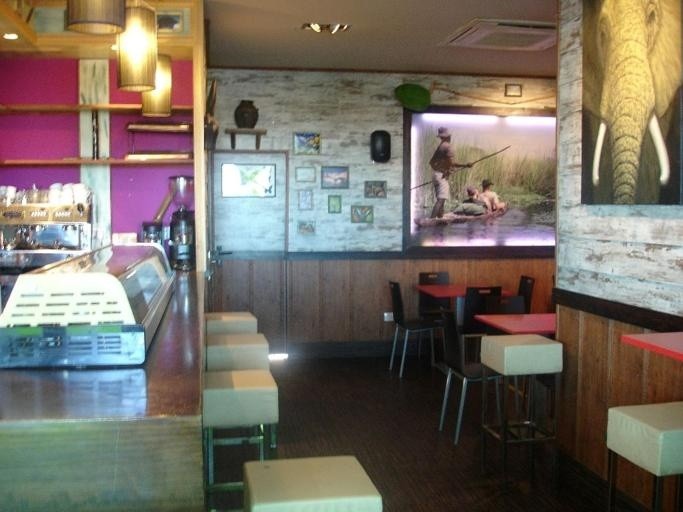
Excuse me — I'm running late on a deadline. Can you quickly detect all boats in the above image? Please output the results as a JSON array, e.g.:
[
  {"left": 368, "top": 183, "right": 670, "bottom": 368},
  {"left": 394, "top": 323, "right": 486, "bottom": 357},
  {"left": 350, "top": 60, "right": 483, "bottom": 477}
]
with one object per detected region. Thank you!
[{"left": 414, "top": 201, "right": 509, "bottom": 228}]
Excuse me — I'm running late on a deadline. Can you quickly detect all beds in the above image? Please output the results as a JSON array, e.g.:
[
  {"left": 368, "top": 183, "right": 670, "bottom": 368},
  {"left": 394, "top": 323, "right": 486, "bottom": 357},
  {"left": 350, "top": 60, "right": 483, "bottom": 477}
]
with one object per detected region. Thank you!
[{"left": 480, "top": 292, "right": 529, "bottom": 419}]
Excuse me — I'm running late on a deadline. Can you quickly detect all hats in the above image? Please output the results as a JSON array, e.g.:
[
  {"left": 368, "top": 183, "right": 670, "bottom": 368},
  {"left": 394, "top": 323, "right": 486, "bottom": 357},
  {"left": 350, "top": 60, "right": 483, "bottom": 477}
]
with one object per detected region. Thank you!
[
  {"left": 483, "top": 179, "right": 493, "bottom": 187},
  {"left": 437, "top": 127, "right": 451, "bottom": 138},
  {"left": 467, "top": 186, "right": 475, "bottom": 194}
]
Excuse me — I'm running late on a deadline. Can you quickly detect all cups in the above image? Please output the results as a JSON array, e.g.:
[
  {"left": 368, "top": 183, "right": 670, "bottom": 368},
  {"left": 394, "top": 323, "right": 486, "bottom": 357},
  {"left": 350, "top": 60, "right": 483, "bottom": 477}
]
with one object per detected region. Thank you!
[
  {"left": 0, "top": 185, "right": 16, "bottom": 204},
  {"left": 48, "top": 182, "right": 88, "bottom": 208}
]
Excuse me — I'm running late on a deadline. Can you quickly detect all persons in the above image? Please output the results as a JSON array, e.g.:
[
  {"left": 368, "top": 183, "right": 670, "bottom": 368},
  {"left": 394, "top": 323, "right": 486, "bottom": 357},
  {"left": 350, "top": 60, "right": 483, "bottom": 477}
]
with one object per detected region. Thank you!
[
  {"left": 425, "top": 125, "right": 473, "bottom": 218},
  {"left": 480, "top": 179, "right": 509, "bottom": 211},
  {"left": 461, "top": 185, "right": 488, "bottom": 214}
]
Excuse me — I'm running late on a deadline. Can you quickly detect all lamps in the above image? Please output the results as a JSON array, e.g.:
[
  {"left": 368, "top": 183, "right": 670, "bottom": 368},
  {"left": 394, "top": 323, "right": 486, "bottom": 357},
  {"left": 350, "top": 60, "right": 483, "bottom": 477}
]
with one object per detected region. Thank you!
[
  {"left": 304, "top": 22, "right": 352, "bottom": 34},
  {"left": 62, "top": 0, "right": 129, "bottom": 37},
  {"left": 139, "top": 50, "right": 175, "bottom": 120},
  {"left": 114, "top": 6, "right": 157, "bottom": 95}
]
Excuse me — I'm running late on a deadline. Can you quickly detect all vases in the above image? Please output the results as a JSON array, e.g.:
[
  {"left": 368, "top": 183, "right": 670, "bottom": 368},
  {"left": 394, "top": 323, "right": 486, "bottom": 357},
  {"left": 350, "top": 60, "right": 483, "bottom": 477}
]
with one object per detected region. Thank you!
[{"left": 232, "top": 99, "right": 258, "bottom": 127}]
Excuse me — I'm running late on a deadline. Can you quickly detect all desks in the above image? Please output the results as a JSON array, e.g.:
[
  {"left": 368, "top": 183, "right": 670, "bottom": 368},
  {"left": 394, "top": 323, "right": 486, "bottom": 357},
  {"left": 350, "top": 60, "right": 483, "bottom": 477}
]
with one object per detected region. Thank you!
[
  {"left": 614, "top": 329, "right": 682, "bottom": 367},
  {"left": 475, "top": 310, "right": 559, "bottom": 446}
]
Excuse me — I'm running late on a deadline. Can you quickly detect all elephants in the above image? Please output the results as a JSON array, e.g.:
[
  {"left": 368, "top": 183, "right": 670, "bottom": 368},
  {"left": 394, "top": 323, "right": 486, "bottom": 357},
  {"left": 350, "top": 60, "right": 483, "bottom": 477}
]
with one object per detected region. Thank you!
[{"left": 584, "top": 0, "right": 680, "bottom": 205}]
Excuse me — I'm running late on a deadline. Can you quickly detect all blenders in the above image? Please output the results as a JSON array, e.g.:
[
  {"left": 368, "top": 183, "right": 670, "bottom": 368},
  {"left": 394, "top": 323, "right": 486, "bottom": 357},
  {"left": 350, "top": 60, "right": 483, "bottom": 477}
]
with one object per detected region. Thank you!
[
  {"left": 140, "top": 220, "right": 162, "bottom": 247},
  {"left": 167, "top": 173, "right": 195, "bottom": 272}
]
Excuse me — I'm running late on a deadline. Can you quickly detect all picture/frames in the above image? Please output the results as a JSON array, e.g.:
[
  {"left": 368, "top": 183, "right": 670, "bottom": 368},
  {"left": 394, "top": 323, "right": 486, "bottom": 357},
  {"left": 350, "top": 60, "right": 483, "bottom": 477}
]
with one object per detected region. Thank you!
[
  {"left": 153, "top": 9, "right": 183, "bottom": 33},
  {"left": 291, "top": 132, "right": 323, "bottom": 155},
  {"left": 294, "top": 166, "right": 317, "bottom": 184},
  {"left": 319, "top": 166, "right": 350, "bottom": 189},
  {"left": 295, "top": 188, "right": 315, "bottom": 212},
  {"left": 218, "top": 161, "right": 277, "bottom": 198},
  {"left": 362, "top": 180, "right": 388, "bottom": 199},
  {"left": 349, "top": 204, "right": 374, "bottom": 224},
  {"left": 297, "top": 219, "right": 317, "bottom": 236},
  {"left": 504, "top": 82, "right": 523, "bottom": 97},
  {"left": 326, "top": 194, "right": 341, "bottom": 214},
  {"left": 399, "top": 104, "right": 558, "bottom": 259}
]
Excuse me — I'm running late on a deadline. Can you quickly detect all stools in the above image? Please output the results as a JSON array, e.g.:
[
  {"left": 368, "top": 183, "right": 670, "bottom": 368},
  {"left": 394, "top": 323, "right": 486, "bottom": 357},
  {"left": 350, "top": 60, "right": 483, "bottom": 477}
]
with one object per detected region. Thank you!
[
  {"left": 201, "top": 328, "right": 272, "bottom": 373},
  {"left": 599, "top": 391, "right": 682, "bottom": 511},
  {"left": 472, "top": 331, "right": 566, "bottom": 494},
  {"left": 236, "top": 452, "right": 385, "bottom": 512},
  {"left": 203, "top": 307, "right": 262, "bottom": 339},
  {"left": 197, "top": 364, "right": 281, "bottom": 512}
]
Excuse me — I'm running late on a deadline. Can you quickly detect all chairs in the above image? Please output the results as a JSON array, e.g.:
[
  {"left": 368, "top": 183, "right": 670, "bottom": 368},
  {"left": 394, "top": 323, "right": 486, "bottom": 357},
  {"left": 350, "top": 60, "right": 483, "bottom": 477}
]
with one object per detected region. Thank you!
[
  {"left": 380, "top": 272, "right": 448, "bottom": 379},
  {"left": 414, "top": 269, "right": 456, "bottom": 369},
  {"left": 433, "top": 299, "right": 522, "bottom": 444},
  {"left": 456, "top": 284, "right": 502, "bottom": 372},
  {"left": 503, "top": 276, "right": 536, "bottom": 317}
]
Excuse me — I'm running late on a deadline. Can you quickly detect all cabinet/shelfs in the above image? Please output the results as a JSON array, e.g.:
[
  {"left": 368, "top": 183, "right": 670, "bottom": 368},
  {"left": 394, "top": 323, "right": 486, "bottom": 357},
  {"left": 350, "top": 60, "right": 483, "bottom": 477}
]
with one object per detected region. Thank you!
[{"left": 0, "top": 98, "right": 197, "bottom": 174}]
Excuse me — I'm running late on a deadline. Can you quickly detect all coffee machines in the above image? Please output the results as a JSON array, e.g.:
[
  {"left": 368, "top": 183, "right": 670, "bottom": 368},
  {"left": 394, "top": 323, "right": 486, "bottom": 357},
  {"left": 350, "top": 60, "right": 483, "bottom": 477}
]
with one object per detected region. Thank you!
[{"left": 0, "top": 202, "right": 91, "bottom": 316}]
